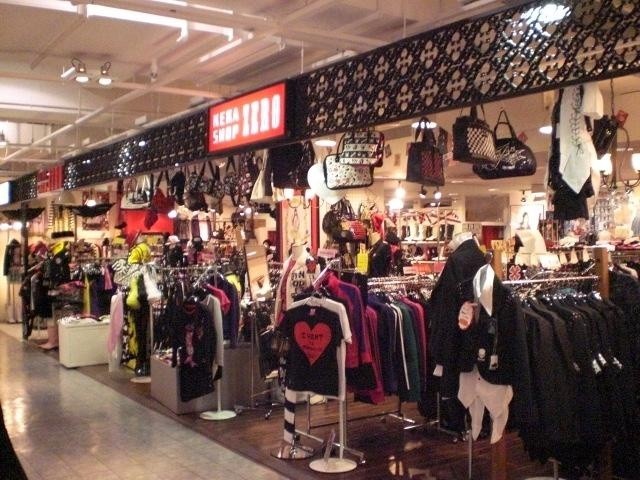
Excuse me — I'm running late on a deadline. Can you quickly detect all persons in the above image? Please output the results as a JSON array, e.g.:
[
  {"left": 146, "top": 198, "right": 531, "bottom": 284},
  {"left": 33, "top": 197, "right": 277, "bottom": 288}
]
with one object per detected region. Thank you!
[
  {"left": 273, "top": 242, "right": 315, "bottom": 366},
  {"left": 426, "top": 232, "right": 485, "bottom": 434},
  {"left": 366, "top": 233, "right": 392, "bottom": 279}
]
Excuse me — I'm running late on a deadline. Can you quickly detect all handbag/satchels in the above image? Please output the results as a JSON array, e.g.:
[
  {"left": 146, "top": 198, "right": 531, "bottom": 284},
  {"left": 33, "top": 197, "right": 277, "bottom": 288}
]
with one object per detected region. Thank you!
[
  {"left": 290, "top": 111, "right": 385, "bottom": 206},
  {"left": 474, "top": 110, "right": 537, "bottom": 180},
  {"left": 120, "top": 156, "right": 286, "bottom": 212},
  {"left": 592, "top": 114, "right": 618, "bottom": 156},
  {"left": 323, "top": 197, "right": 368, "bottom": 244},
  {"left": 406, "top": 117, "right": 445, "bottom": 188},
  {"left": 452, "top": 88, "right": 498, "bottom": 166}
]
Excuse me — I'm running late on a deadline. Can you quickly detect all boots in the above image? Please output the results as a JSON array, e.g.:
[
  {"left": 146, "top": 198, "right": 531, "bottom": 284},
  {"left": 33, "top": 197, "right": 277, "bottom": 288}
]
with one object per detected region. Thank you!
[{"left": 401, "top": 224, "right": 454, "bottom": 242}]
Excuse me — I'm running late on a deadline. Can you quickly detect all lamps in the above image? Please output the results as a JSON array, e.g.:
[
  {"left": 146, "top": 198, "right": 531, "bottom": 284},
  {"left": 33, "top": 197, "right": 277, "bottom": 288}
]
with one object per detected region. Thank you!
[{"left": 60, "top": 57, "right": 113, "bottom": 86}]
